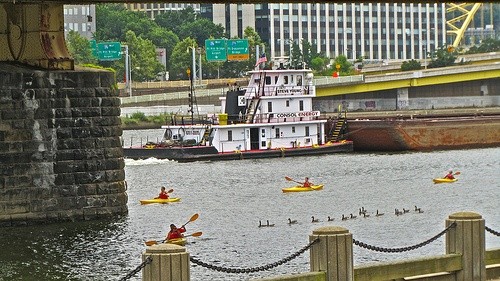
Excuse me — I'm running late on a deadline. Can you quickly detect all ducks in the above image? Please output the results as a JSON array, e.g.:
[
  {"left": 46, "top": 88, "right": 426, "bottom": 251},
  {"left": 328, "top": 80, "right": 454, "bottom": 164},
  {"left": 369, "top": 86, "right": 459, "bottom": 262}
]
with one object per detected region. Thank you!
[{"left": 257, "top": 205, "right": 424, "bottom": 228}]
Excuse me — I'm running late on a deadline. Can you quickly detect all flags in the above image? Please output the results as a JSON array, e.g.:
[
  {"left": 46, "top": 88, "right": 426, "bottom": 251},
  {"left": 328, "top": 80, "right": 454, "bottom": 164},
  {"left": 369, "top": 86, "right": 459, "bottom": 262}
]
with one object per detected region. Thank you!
[{"left": 255, "top": 53, "right": 267, "bottom": 66}]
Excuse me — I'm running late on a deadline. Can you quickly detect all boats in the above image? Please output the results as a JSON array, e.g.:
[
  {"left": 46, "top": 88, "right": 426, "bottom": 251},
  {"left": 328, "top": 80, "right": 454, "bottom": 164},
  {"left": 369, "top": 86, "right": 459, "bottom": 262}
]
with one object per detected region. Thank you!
[
  {"left": 145, "top": 238, "right": 187, "bottom": 246},
  {"left": 122, "top": 61, "right": 353, "bottom": 162},
  {"left": 433, "top": 178, "right": 459, "bottom": 184},
  {"left": 139, "top": 197, "right": 181, "bottom": 205},
  {"left": 281, "top": 183, "right": 324, "bottom": 192}
]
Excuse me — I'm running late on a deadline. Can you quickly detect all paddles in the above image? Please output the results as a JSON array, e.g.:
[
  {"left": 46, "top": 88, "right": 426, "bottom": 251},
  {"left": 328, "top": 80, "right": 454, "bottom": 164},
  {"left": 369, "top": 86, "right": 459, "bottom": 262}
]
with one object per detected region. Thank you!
[
  {"left": 164, "top": 213, "right": 199, "bottom": 240},
  {"left": 145, "top": 231, "right": 202, "bottom": 246},
  {"left": 154, "top": 189, "right": 173, "bottom": 199},
  {"left": 284, "top": 176, "right": 304, "bottom": 185},
  {"left": 446, "top": 172, "right": 460, "bottom": 179}
]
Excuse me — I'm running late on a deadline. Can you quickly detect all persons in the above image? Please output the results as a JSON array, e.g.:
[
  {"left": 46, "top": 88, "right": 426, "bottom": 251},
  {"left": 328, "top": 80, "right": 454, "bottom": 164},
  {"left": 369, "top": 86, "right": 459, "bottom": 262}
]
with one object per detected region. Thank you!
[
  {"left": 302, "top": 177, "right": 312, "bottom": 187},
  {"left": 166, "top": 224, "right": 187, "bottom": 241},
  {"left": 445, "top": 170, "right": 455, "bottom": 179},
  {"left": 159, "top": 186, "right": 169, "bottom": 199}
]
